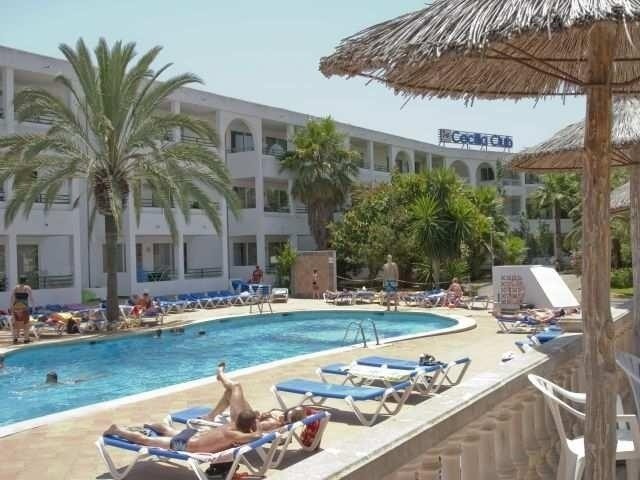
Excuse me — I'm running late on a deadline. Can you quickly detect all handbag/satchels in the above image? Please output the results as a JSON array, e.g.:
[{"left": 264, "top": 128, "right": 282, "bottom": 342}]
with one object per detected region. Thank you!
[{"left": 284, "top": 405, "right": 331, "bottom": 452}]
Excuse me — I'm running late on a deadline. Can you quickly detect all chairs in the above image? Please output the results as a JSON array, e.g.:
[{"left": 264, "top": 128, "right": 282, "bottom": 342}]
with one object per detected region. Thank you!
[
  {"left": 321, "top": 288, "right": 491, "bottom": 310},
  {"left": 98, "top": 423, "right": 280, "bottom": 479},
  {"left": 495, "top": 318, "right": 562, "bottom": 334},
  {"left": 0, "top": 274, "right": 290, "bottom": 340},
  {"left": 527, "top": 348, "right": 640, "bottom": 479},
  {"left": 320, "top": 362, "right": 445, "bottom": 402},
  {"left": 272, "top": 378, "right": 412, "bottom": 427},
  {"left": 172, "top": 406, "right": 332, "bottom": 468},
  {"left": 352, "top": 356, "right": 472, "bottom": 394}
]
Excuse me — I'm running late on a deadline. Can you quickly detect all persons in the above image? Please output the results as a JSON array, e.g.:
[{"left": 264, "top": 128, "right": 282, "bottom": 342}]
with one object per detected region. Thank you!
[
  {"left": 11, "top": 275, "right": 35, "bottom": 344},
  {"left": 45, "top": 372, "right": 90, "bottom": 385},
  {"left": 128, "top": 293, "right": 154, "bottom": 309},
  {"left": 311, "top": 269, "right": 320, "bottom": 299},
  {"left": 103, "top": 362, "right": 304, "bottom": 453},
  {"left": 247, "top": 266, "right": 263, "bottom": 283},
  {"left": 447, "top": 278, "right": 462, "bottom": 307},
  {"left": 384, "top": 254, "right": 399, "bottom": 311},
  {"left": 153, "top": 328, "right": 206, "bottom": 338},
  {"left": 492, "top": 308, "right": 565, "bottom": 325},
  {"left": 0, "top": 354, "right": 6, "bottom": 368}
]
